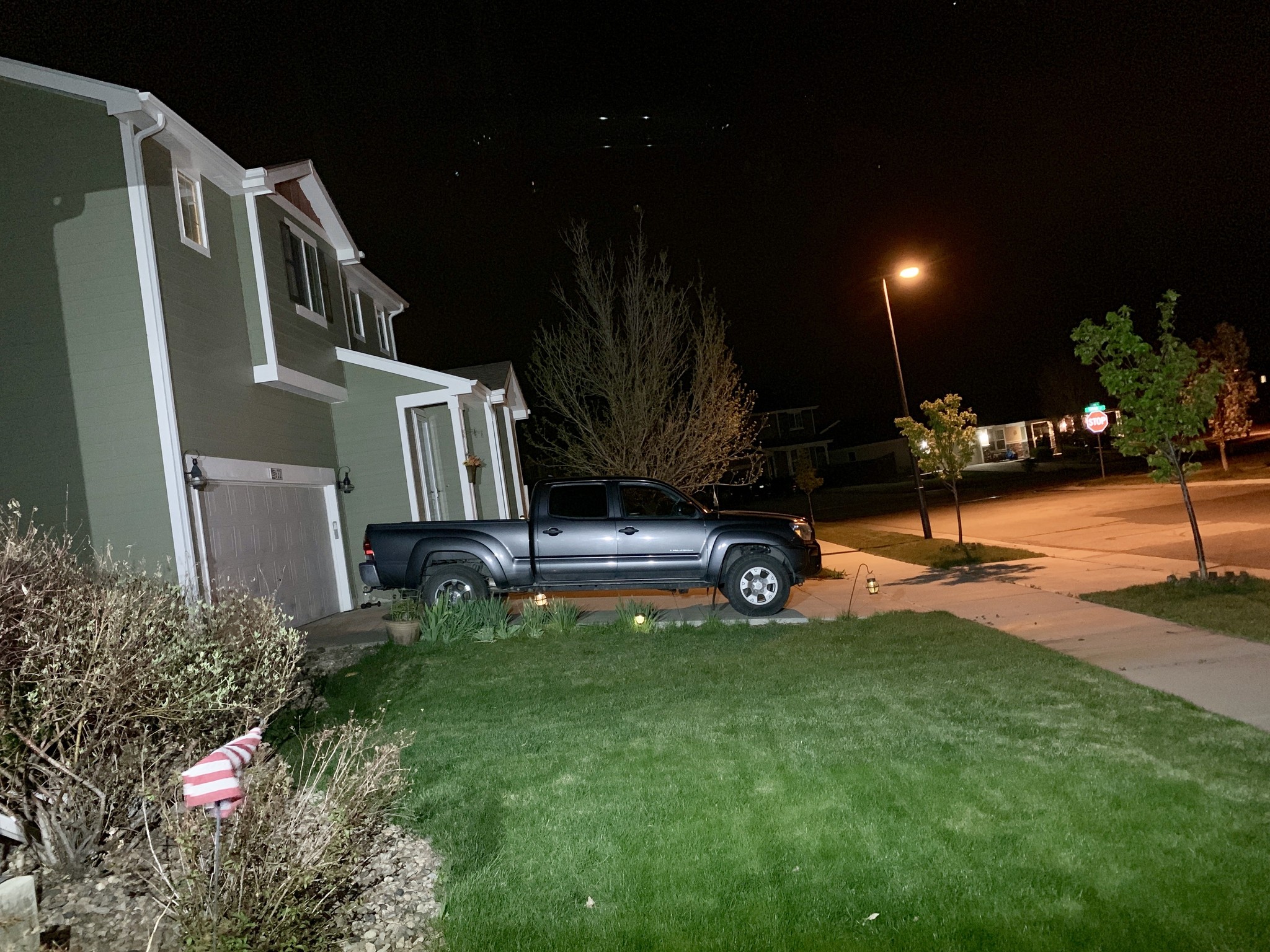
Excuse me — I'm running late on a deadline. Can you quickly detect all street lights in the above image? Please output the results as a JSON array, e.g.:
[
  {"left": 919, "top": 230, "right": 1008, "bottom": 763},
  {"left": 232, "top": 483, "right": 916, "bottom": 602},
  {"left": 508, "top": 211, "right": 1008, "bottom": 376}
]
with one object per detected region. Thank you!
[{"left": 881, "top": 267, "right": 932, "bottom": 539}]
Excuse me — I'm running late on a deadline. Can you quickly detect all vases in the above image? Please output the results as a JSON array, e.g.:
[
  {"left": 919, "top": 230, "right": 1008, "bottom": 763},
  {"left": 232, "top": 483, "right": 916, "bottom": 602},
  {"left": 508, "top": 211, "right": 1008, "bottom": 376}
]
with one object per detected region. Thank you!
[{"left": 465, "top": 465, "right": 476, "bottom": 483}]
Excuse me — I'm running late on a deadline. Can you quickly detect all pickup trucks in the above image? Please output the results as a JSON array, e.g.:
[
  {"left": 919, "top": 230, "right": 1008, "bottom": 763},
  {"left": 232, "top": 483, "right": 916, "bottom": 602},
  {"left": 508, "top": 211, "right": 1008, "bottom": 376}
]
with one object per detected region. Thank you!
[{"left": 358, "top": 476, "right": 822, "bottom": 616}]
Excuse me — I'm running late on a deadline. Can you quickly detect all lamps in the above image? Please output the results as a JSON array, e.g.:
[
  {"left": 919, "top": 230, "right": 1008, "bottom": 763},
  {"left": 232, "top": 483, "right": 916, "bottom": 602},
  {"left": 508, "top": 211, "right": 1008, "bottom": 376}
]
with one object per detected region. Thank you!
[
  {"left": 635, "top": 613, "right": 646, "bottom": 624},
  {"left": 336, "top": 466, "right": 354, "bottom": 494},
  {"left": 865, "top": 578, "right": 879, "bottom": 595},
  {"left": 183, "top": 448, "right": 208, "bottom": 489},
  {"left": 534, "top": 589, "right": 547, "bottom": 605}
]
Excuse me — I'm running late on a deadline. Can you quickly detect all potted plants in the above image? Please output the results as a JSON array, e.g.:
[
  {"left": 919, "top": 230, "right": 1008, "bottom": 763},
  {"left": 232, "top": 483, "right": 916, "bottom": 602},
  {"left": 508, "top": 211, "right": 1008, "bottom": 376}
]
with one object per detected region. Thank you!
[{"left": 382, "top": 599, "right": 420, "bottom": 643}]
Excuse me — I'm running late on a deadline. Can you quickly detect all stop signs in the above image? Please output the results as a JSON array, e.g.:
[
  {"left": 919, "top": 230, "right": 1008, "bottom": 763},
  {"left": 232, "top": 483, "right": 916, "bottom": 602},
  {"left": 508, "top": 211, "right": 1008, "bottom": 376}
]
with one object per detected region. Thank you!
[{"left": 1086, "top": 411, "right": 1109, "bottom": 433}]
